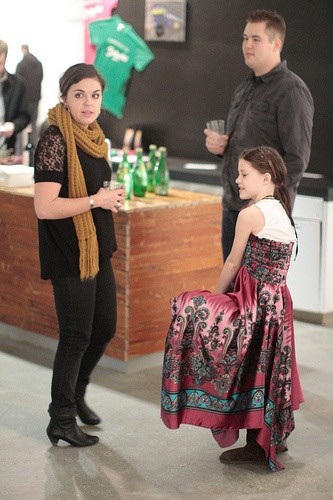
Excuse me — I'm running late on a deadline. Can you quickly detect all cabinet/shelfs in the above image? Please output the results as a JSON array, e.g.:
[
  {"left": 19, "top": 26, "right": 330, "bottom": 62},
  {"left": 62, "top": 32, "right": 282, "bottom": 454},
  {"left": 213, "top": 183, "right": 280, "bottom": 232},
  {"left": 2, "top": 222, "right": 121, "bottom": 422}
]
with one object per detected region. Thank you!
[{"left": 166, "top": 157, "right": 333, "bottom": 326}]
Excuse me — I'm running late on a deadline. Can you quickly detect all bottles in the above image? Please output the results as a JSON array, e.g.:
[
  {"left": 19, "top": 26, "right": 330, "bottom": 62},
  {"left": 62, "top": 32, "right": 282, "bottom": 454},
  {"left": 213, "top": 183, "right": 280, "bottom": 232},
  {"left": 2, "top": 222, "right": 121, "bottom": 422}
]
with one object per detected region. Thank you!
[
  {"left": 22, "top": 132, "right": 35, "bottom": 167},
  {"left": 115, "top": 147, "right": 131, "bottom": 200},
  {"left": 154, "top": 146, "right": 170, "bottom": 196},
  {"left": 147, "top": 145, "right": 158, "bottom": 193},
  {"left": 132, "top": 148, "right": 147, "bottom": 198}
]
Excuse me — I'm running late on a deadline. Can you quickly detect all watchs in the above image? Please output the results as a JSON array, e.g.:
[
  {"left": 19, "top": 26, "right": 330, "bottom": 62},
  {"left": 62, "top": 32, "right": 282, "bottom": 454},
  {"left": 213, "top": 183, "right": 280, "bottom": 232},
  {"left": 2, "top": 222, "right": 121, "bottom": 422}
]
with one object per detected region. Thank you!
[{"left": 89, "top": 196, "right": 94, "bottom": 209}]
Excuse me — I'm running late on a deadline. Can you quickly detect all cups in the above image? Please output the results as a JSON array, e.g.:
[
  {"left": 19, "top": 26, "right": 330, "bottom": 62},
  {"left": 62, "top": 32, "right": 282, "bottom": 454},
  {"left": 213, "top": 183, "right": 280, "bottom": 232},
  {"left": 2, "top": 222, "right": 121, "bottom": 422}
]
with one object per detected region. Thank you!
[
  {"left": 205, "top": 119, "right": 226, "bottom": 135},
  {"left": 103, "top": 181, "right": 122, "bottom": 210}
]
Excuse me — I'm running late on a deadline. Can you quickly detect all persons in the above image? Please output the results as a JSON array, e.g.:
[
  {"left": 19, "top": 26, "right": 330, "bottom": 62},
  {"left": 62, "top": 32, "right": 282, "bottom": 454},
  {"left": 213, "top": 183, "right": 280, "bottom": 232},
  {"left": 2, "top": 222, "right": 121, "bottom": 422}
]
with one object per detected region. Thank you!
[
  {"left": 203, "top": 9, "right": 314, "bottom": 265},
  {"left": 160, "top": 147, "right": 304, "bottom": 472},
  {"left": 0, "top": 40, "right": 43, "bottom": 157},
  {"left": 33, "top": 63, "right": 126, "bottom": 446}
]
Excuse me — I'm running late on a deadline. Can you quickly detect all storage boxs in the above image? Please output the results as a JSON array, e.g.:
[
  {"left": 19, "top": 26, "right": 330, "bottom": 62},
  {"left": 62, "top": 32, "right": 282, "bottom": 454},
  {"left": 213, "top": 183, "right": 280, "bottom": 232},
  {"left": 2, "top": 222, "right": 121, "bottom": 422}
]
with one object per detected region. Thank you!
[{"left": 0, "top": 165, "right": 34, "bottom": 187}]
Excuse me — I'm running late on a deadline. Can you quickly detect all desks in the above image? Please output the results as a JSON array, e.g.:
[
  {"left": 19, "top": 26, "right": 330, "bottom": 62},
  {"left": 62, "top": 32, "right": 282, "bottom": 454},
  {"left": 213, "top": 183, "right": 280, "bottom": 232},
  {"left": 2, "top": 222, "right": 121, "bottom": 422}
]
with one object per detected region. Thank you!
[{"left": 0, "top": 186, "right": 223, "bottom": 375}]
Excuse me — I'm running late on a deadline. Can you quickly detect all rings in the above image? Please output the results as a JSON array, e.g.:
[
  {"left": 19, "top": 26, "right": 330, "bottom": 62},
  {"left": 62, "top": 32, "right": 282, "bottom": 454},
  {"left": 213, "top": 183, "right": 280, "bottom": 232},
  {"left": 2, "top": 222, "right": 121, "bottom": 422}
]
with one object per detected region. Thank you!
[{"left": 116, "top": 203, "right": 121, "bottom": 208}]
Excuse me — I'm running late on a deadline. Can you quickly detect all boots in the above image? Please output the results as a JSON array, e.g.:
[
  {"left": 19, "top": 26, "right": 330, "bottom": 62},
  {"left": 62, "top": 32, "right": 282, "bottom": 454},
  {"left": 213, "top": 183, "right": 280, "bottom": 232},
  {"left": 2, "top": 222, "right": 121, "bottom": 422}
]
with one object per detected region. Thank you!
[
  {"left": 75, "top": 382, "right": 100, "bottom": 425},
  {"left": 46, "top": 402, "right": 98, "bottom": 447}
]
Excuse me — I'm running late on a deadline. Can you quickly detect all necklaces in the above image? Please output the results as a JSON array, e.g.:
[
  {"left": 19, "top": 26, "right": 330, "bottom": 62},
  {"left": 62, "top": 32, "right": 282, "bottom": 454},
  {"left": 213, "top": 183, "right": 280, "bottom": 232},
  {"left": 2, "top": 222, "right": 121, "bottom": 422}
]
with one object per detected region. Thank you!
[{"left": 262, "top": 195, "right": 274, "bottom": 199}]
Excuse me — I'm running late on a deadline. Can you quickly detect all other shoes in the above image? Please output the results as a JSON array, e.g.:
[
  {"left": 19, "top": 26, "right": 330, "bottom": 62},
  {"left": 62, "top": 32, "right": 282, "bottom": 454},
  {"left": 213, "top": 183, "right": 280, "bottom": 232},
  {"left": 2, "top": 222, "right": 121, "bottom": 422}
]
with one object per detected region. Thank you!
[{"left": 219, "top": 428, "right": 267, "bottom": 465}]
